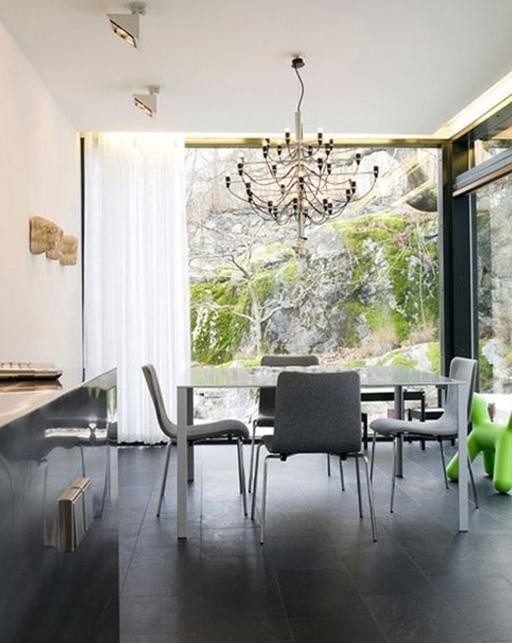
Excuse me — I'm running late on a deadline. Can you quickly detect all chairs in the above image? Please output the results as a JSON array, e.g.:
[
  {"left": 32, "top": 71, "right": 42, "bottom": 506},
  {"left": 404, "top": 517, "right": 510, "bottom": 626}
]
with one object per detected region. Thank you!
[
  {"left": 369, "top": 356, "right": 478, "bottom": 513},
  {"left": 250, "top": 371, "right": 378, "bottom": 545},
  {"left": 250, "top": 355, "right": 319, "bottom": 494},
  {"left": 142, "top": 364, "right": 249, "bottom": 518},
  {"left": 408, "top": 385, "right": 455, "bottom": 450}
]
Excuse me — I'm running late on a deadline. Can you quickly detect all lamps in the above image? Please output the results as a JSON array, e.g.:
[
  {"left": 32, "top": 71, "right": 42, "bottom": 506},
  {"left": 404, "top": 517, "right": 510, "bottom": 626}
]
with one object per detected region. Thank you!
[
  {"left": 134, "top": 86, "right": 158, "bottom": 115},
  {"left": 107, "top": 3, "right": 147, "bottom": 49},
  {"left": 226, "top": 57, "right": 377, "bottom": 255}
]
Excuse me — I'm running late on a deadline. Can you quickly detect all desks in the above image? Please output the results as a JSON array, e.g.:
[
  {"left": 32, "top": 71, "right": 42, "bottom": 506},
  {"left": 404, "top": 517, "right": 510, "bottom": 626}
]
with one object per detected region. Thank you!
[
  {"left": 175, "top": 364, "right": 469, "bottom": 539},
  {"left": 361, "top": 386, "right": 426, "bottom": 449}
]
